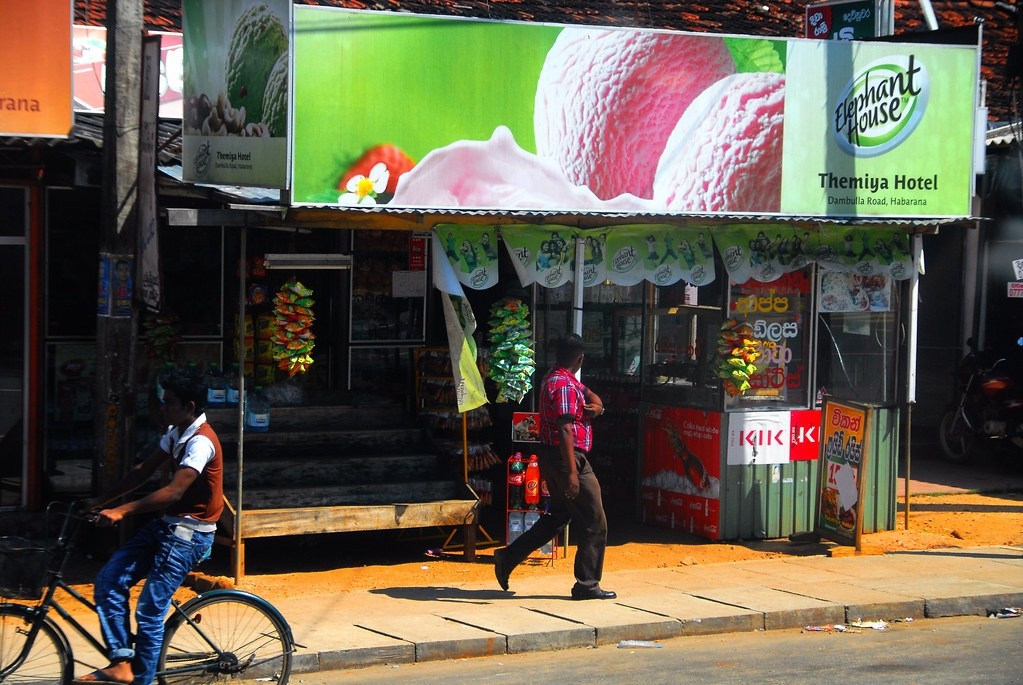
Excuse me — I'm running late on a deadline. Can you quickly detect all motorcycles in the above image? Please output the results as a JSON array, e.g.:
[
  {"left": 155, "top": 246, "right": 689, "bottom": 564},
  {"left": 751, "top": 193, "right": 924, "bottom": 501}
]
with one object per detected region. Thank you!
[{"left": 940, "top": 335, "right": 1023, "bottom": 462}]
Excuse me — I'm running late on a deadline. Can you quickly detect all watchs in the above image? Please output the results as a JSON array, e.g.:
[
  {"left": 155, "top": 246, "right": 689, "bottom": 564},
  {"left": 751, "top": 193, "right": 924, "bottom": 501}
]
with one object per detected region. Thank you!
[{"left": 598, "top": 404, "right": 605, "bottom": 416}]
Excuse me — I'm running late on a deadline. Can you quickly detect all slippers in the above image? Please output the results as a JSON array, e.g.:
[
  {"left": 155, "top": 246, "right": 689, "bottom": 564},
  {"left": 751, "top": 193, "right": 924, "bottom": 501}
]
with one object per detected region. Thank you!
[{"left": 72, "top": 670, "right": 126, "bottom": 685}]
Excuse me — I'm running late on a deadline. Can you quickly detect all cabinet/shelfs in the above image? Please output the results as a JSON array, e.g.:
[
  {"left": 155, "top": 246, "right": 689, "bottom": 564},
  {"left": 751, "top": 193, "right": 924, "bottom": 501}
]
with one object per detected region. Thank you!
[
  {"left": 198, "top": 400, "right": 482, "bottom": 585},
  {"left": 412, "top": 345, "right": 503, "bottom": 550}
]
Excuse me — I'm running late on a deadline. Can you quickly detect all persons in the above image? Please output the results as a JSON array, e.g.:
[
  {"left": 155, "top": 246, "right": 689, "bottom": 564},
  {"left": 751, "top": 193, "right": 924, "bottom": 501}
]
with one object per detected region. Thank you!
[
  {"left": 749, "top": 231, "right": 809, "bottom": 268},
  {"left": 535, "top": 233, "right": 576, "bottom": 272},
  {"left": 494, "top": 332, "right": 619, "bottom": 599},
  {"left": 695, "top": 233, "right": 713, "bottom": 259},
  {"left": 75, "top": 368, "right": 222, "bottom": 685},
  {"left": 677, "top": 238, "right": 699, "bottom": 270},
  {"left": 874, "top": 238, "right": 896, "bottom": 265},
  {"left": 460, "top": 240, "right": 480, "bottom": 273},
  {"left": 513, "top": 414, "right": 538, "bottom": 440},
  {"left": 892, "top": 232, "right": 910, "bottom": 257},
  {"left": 660, "top": 230, "right": 678, "bottom": 265},
  {"left": 641, "top": 235, "right": 660, "bottom": 265},
  {"left": 584, "top": 234, "right": 607, "bottom": 265},
  {"left": 857, "top": 230, "right": 875, "bottom": 260},
  {"left": 839, "top": 233, "right": 857, "bottom": 257},
  {"left": 478, "top": 233, "right": 497, "bottom": 262},
  {"left": 98, "top": 259, "right": 104, "bottom": 279},
  {"left": 114, "top": 261, "right": 131, "bottom": 282},
  {"left": 438, "top": 227, "right": 461, "bottom": 263}
]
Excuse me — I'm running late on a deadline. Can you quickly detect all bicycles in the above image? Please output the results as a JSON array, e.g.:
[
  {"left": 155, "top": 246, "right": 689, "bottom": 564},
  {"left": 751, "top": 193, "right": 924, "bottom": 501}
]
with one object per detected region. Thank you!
[{"left": 0, "top": 494, "right": 298, "bottom": 685}]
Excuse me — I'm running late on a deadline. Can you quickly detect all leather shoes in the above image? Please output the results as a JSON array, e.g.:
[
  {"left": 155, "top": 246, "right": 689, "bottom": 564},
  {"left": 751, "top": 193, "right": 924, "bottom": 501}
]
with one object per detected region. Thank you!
[
  {"left": 494, "top": 549, "right": 509, "bottom": 591},
  {"left": 571, "top": 583, "right": 617, "bottom": 601}
]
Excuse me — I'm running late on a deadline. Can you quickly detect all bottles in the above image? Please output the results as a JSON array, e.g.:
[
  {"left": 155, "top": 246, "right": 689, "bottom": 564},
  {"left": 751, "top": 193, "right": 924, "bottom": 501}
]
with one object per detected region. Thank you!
[
  {"left": 524, "top": 505, "right": 540, "bottom": 533},
  {"left": 206, "top": 362, "right": 225, "bottom": 408},
  {"left": 539, "top": 472, "right": 551, "bottom": 510},
  {"left": 244, "top": 385, "right": 270, "bottom": 431},
  {"left": 525, "top": 455, "right": 540, "bottom": 509},
  {"left": 540, "top": 537, "right": 555, "bottom": 555},
  {"left": 509, "top": 452, "right": 525, "bottom": 509},
  {"left": 157, "top": 362, "right": 196, "bottom": 404},
  {"left": 508, "top": 503, "right": 524, "bottom": 545},
  {"left": 225, "top": 363, "right": 247, "bottom": 408}
]
